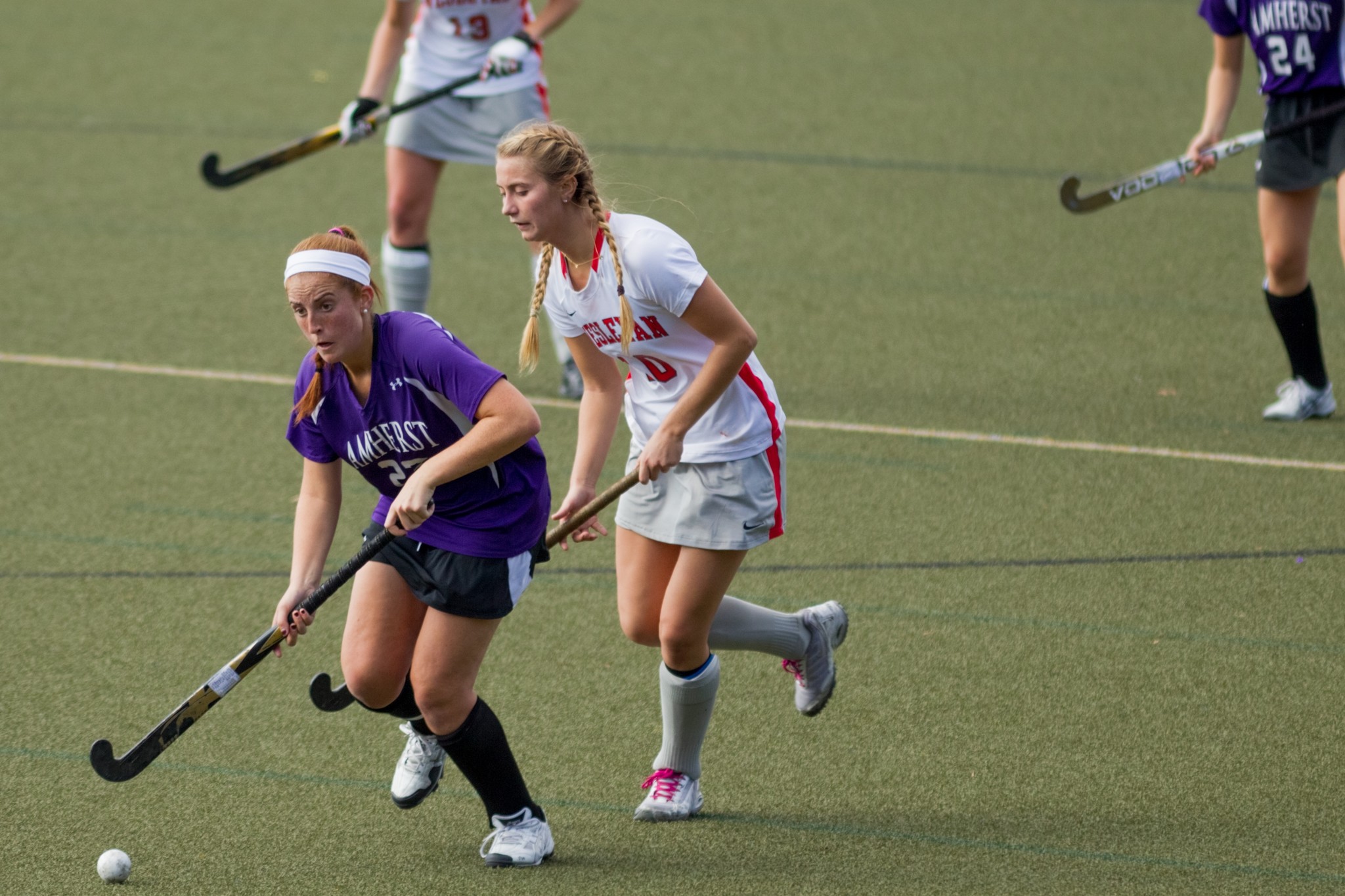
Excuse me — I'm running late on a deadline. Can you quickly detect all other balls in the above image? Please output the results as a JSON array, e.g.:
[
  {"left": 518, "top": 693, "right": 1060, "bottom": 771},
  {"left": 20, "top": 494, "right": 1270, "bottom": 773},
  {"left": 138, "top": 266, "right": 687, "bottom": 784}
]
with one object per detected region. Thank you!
[{"left": 97, "top": 848, "right": 132, "bottom": 883}]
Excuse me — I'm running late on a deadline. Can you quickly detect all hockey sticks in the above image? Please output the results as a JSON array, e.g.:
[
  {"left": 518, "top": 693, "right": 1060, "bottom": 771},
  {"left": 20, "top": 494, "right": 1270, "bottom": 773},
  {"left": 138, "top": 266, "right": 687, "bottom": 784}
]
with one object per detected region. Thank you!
[
  {"left": 306, "top": 463, "right": 643, "bottom": 714},
  {"left": 198, "top": 60, "right": 523, "bottom": 191},
  {"left": 1059, "top": 99, "right": 1345, "bottom": 217},
  {"left": 88, "top": 496, "right": 433, "bottom": 784}
]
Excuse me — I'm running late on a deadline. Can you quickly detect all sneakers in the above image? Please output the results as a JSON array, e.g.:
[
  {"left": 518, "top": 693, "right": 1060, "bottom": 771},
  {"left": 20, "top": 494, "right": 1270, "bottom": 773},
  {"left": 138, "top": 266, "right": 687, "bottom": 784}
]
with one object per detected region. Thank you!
[
  {"left": 1264, "top": 376, "right": 1337, "bottom": 419},
  {"left": 785, "top": 599, "right": 848, "bottom": 719},
  {"left": 634, "top": 767, "right": 705, "bottom": 822},
  {"left": 390, "top": 722, "right": 447, "bottom": 808},
  {"left": 479, "top": 807, "right": 555, "bottom": 867}
]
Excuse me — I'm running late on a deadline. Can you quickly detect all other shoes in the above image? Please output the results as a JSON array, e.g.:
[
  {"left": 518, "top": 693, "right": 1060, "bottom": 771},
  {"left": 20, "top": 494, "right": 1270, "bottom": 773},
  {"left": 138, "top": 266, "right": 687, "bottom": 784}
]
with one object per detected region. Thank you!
[{"left": 560, "top": 358, "right": 587, "bottom": 400}]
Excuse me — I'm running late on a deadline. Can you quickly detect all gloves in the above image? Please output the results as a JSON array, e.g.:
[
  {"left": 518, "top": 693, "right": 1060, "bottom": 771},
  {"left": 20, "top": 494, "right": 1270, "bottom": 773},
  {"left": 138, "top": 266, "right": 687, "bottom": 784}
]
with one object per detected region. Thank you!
[
  {"left": 478, "top": 35, "right": 531, "bottom": 80},
  {"left": 334, "top": 97, "right": 384, "bottom": 146}
]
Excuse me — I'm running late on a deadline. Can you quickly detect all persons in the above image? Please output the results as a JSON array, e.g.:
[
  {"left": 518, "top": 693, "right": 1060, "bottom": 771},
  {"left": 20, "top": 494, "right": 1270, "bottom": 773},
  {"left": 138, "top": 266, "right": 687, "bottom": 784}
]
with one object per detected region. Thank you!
[
  {"left": 340, "top": 0, "right": 584, "bottom": 398},
  {"left": 496, "top": 119, "right": 848, "bottom": 821},
  {"left": 271, "top": 227, "right": 555, "bottom": 869},
  {"left": 1189, "top": 0, "right": 1345, "bottom": 420}
]
